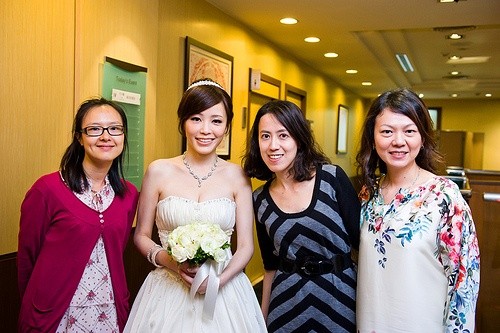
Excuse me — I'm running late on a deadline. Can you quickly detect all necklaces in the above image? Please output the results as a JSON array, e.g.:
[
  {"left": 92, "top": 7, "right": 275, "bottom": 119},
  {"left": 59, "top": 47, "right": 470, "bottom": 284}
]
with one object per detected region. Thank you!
[
  {"left": 408, "top": 167, "right": 420, "bottom": 188},
  {"left": 183, "top": 151, "right": 219, "bottom": 188}
]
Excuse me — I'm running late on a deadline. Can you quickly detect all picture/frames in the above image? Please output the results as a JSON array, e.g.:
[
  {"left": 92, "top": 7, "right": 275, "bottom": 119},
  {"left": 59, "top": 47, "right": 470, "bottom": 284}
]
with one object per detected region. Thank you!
[
  {"left": 182, "top": 36, "right": 234, "bottom": 161},
  {"left": 335, "top": 104, "right": 349, "bottom": 155}
]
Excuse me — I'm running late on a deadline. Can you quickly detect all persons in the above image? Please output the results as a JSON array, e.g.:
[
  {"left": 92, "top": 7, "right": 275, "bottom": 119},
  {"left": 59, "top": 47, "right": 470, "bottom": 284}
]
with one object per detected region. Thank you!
[
  {"left": 356, "top": 89, "right": 480, "bottom": 333},
  {"left": 243, "top": 100, "right": 361, "bottom": 333},
  {"left": 19, "top": 98, "right": 140, "bottom": 333},
  {"left": 121, "top": 78, "right": 268, "bottom": 333}
]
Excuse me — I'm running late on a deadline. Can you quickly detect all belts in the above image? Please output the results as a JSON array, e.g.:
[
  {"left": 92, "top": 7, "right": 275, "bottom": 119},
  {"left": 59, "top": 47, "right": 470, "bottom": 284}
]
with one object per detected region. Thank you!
[{"left": 276, "top": 251, "right": 353, "bottom": 276}]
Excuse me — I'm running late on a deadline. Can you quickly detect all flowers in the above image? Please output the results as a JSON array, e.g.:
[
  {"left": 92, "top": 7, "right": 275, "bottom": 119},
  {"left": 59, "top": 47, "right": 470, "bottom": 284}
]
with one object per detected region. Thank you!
[{"left": 165, "top": 223, "right": 232, "bottom": 271}]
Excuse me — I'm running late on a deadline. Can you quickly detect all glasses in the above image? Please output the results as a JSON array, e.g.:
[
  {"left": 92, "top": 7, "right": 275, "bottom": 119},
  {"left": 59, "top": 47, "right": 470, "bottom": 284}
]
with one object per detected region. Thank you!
[{"left": 80, "top": 125, "right": 125, "bottom": 136}]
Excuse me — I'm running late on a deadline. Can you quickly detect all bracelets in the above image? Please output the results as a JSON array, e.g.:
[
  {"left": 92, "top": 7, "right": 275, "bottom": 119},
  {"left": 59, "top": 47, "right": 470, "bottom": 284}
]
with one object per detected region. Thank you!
[{"left": 147, "top": 244, "right": 165, "bottom": 267}]
